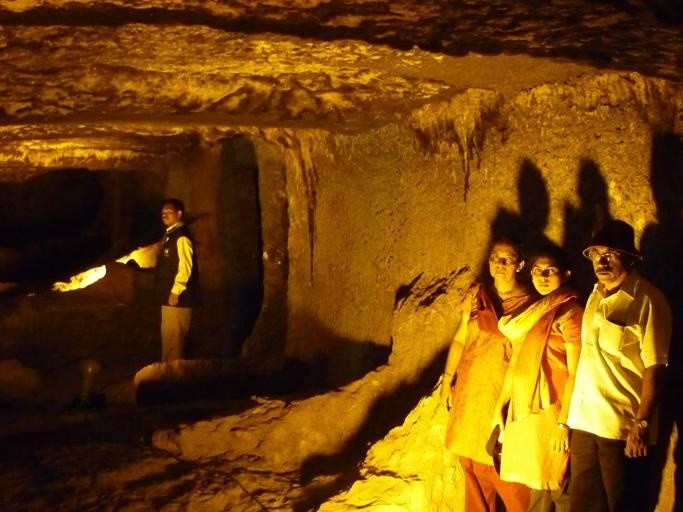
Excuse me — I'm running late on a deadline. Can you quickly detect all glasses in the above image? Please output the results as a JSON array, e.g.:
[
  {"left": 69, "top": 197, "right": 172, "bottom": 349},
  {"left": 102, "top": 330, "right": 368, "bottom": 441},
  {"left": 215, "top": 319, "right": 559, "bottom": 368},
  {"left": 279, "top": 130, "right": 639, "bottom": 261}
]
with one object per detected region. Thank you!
[{"left": 587, "top": 249, "right": 626, "bottom": 260}]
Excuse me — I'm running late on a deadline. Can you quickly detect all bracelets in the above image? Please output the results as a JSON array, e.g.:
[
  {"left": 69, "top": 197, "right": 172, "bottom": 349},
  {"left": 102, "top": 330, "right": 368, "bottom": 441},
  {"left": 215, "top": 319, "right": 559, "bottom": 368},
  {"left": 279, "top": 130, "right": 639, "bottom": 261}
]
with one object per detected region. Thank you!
[
  {"left": 556, "top": 423, "right": 569, "bottom": 431},
  {"left": 442, "top": 372, "right": 452, "bottom": 378}
]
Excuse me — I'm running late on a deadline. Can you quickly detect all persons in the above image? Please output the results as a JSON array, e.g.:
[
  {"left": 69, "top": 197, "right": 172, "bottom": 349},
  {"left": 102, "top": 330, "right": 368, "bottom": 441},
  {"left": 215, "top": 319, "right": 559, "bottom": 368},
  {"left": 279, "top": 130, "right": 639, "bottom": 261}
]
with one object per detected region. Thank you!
[
  {"left": 490, "top": 255, "right": 585, "bottom": 512},
  {"left": 569, "top": 219, "right": 674, "bottom": 512},
  {"left": 157, "top": 198, "right": 197, "bottom": 362},
  {"left": 440, "top": 237, "right": 535, "bottom": 511}
]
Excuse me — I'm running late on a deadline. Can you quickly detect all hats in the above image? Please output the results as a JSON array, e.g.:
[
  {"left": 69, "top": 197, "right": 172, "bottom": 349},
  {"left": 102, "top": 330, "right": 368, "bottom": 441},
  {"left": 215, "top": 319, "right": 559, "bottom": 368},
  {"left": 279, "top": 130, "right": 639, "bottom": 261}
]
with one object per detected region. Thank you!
[{"left": 581, "top": 218, "right": 644, "bottom": 262}]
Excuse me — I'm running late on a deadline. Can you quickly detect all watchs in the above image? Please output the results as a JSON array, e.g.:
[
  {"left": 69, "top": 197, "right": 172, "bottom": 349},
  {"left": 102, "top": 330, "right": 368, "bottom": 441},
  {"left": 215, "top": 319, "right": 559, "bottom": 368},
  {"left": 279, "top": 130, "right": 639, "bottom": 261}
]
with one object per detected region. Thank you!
[{"left": 633, "top": 418, "right": 649, "bottom": 430}]
